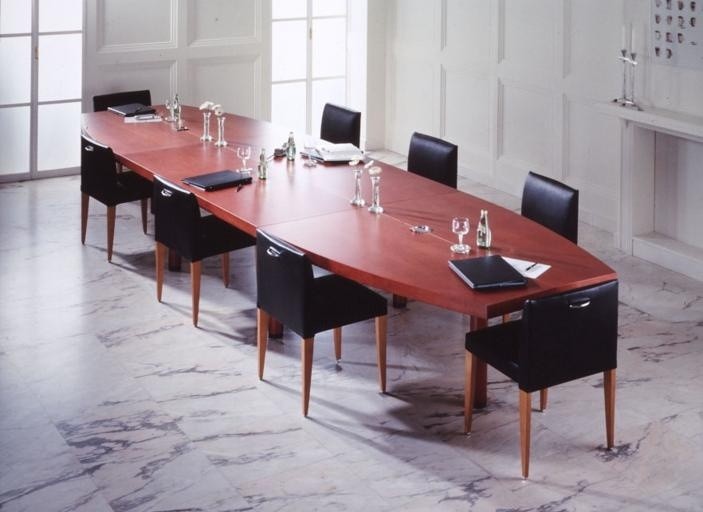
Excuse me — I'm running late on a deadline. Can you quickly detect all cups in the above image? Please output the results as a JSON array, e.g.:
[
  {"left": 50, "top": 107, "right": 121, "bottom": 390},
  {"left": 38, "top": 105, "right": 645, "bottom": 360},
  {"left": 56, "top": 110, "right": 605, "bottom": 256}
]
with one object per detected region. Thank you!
[
  {"left": 303, "top": 138, "right": 318, "bottom": 167},
  {"left": 237, "top": 145, "right": 252, "bottom": 172},
  {"left": 166, "top": 99, "right": 177, "bottom": 122},
  {"left": 450, "top": 218, "right": 470, "bottom": 255}
]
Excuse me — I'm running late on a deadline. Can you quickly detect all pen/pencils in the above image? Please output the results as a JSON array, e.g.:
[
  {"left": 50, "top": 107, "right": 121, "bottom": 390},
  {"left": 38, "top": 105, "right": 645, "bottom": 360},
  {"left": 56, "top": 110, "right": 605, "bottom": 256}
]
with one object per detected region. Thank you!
[
  {"left": 237, "top": 183, "right": 243, "bottom": 191},
  {"left": 136, "top": 116, "right": 158, "bottom": 120},
  {"left": 526, "top": 262, "right": 537, "bottom": 271}
]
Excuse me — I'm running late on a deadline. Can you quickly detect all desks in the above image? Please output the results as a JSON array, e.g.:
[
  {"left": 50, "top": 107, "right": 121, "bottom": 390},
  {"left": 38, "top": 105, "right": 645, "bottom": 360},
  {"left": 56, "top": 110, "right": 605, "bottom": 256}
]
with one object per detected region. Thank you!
[{"left": 81, "top": 100, "right": 618, "bottom": 410}]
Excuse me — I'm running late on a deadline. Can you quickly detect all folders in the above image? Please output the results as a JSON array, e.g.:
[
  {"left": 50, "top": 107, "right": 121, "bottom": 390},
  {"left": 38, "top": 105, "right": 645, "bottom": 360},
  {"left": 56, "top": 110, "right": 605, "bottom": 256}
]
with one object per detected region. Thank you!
[{"left": 181, "top": 168, "right": 253, "bottom": 193}]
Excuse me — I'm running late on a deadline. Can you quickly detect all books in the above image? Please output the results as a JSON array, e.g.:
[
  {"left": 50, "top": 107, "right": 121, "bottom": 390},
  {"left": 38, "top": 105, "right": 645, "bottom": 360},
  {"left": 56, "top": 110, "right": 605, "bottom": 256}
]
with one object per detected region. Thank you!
[
  {"left": 300, "top": 140, "right": 366, "bottom": 163},
  {"left": 183, "top": 170, "right": 254, "bottom": 191}
]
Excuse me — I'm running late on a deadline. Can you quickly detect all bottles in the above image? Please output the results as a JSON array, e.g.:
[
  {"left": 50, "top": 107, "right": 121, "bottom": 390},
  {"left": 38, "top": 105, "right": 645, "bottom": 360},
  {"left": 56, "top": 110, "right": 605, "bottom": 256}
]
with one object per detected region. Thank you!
[
  {"left": 172, "top": 94, "right": 180, "bottom": 122},
  {"left": 258, "top": 149, "right": 267, "bottom": 180},
  {"left": 286, "top": 132, "right": 296, "bottom": 161},
  {"left": 476, "top": 209, "right": 492, "bottom": 248}
]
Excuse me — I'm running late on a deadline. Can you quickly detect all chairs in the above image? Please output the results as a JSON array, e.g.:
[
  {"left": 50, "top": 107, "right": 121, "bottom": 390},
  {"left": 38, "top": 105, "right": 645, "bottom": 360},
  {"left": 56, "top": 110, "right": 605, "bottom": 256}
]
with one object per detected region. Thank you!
[
  {"left": 521, "top": 171, "right": 579, "bottom": 246},
  {"left": 93, "top": 89, "right": 152, "bottom": 114},
  {"left": 320, "top": 103, "right": 362, "bottom": 152},
  {"left": 407, "top": 131, "right": 457, "bottom": 191},
  {"left": 149, "top": 174, "right": 258, "bottom": 326},
  {"left": 255, "top": 226, "right": 389, "bottom": 414},
  {"left": 463, "top": 279, "right": 619, "bottom": 477},
  {"left": 81, "top": 134, "right": 154, "bottom": 259}
]
